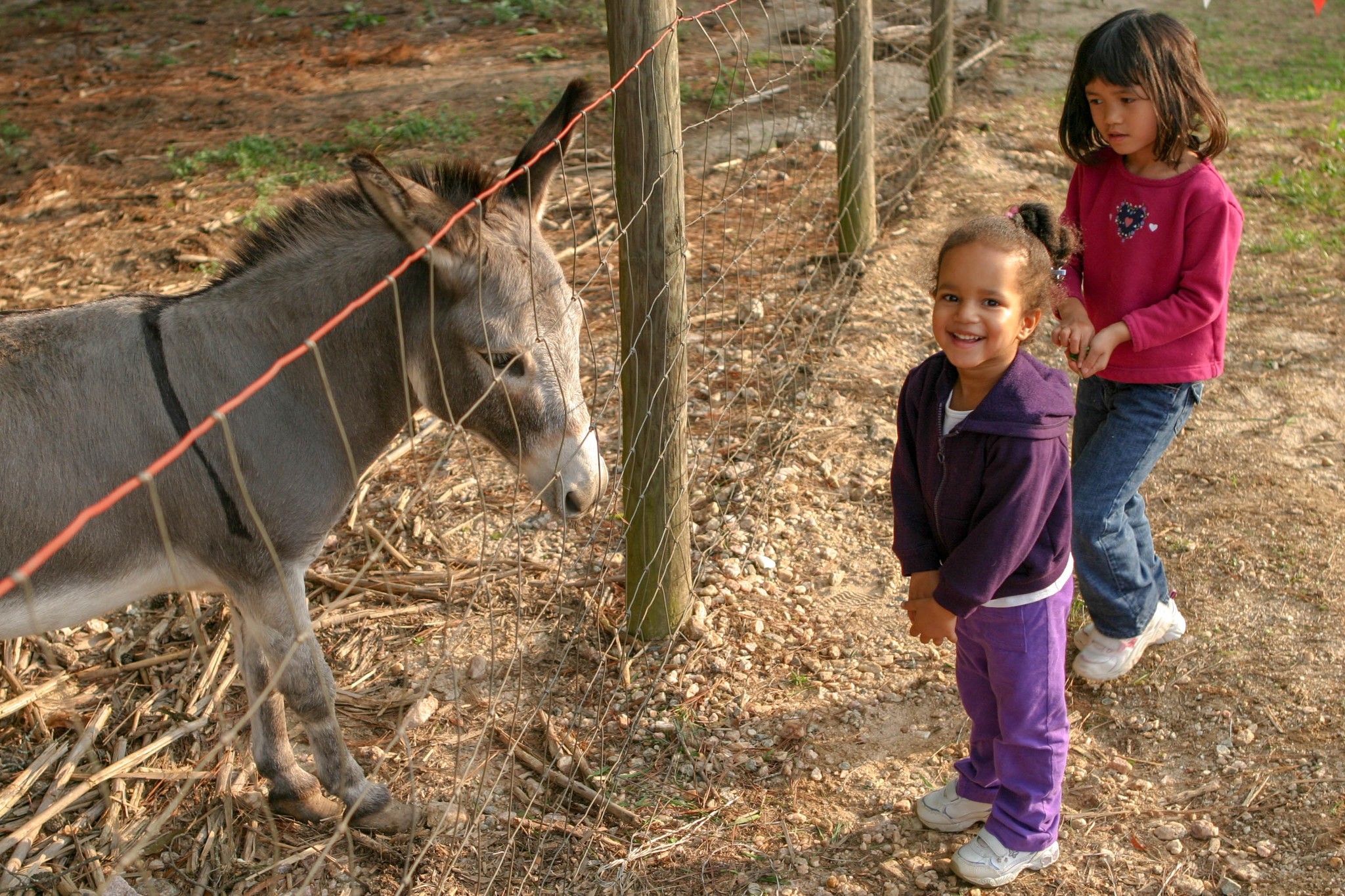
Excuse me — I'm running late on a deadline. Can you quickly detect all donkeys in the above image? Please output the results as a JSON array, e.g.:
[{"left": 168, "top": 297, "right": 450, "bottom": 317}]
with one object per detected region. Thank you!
[{"left": 0, "top": 75, "right": 610, "bottom": 838}]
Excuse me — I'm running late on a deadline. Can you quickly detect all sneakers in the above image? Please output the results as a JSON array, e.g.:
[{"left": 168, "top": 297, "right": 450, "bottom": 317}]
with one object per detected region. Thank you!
[
  {"left": 1074, "top": 590, "right": 1186, "bottom": 651},
  {"left": 948, "top": 826, "right": 1059, "bottom": 889},
  {"left": 1072, "top": 600, "right": 1172, "bottom": 681},
  {"left": 914, "top": 778, "right": 993, "bottom": 832}
]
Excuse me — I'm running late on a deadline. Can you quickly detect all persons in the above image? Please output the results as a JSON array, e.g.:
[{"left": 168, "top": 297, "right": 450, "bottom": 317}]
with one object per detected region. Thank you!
[
  {"left": 890, "top": 197, "right": 1083, "bottom": 888},
  {"left": 1050, "top": 7, "right": 1244, "bottom": 681}
]
